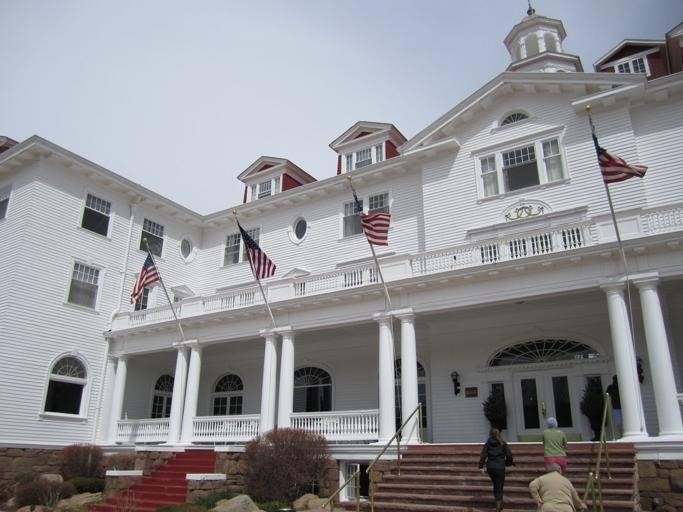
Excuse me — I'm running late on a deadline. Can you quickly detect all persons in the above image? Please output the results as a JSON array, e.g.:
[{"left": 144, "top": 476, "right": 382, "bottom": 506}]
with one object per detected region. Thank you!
[
  {"left": 605, "top": 375, "right": 621, "bottom": 435},
  {"left": 478, "top": 428, "right": 513, "bottom": 511},
  {"left": 528, "top": 462, "right": 588, "bottom": 511},
  {"left": 539, "top": 417, "right": 568, "bottom": 474}
]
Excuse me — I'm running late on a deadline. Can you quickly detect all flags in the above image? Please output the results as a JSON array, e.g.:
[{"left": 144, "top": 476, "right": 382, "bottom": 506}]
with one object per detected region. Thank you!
[
  {"left": 591, "top": 135, "right": 647, "bottom": 185},
  {"left": 237, "top": 225, "right": 276, "bottom": 280},
  {"left": 127, "top": 255, "right": 158, "bottom": 305},
  {"left": 351, "top": 191, "right": 391, "bottom": 247}
]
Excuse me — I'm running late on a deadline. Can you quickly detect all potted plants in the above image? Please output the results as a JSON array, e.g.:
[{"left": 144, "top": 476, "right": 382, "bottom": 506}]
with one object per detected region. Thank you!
[
  {"left": 580, "top": 377, "right": 607, "bottom": 441},
  {"left": 481, "top": 387, "right": 507, "bottom": 435}
]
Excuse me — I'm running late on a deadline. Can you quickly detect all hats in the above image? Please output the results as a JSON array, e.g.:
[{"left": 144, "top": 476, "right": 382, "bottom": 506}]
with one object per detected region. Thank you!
[{"left": 546, "top": 418, "right": 558, "bottom": 428}]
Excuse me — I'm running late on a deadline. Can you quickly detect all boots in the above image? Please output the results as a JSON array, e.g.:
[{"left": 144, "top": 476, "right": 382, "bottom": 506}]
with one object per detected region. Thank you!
[{"left": 495, "top": 500, "right": 503, "bottom": 512}]
